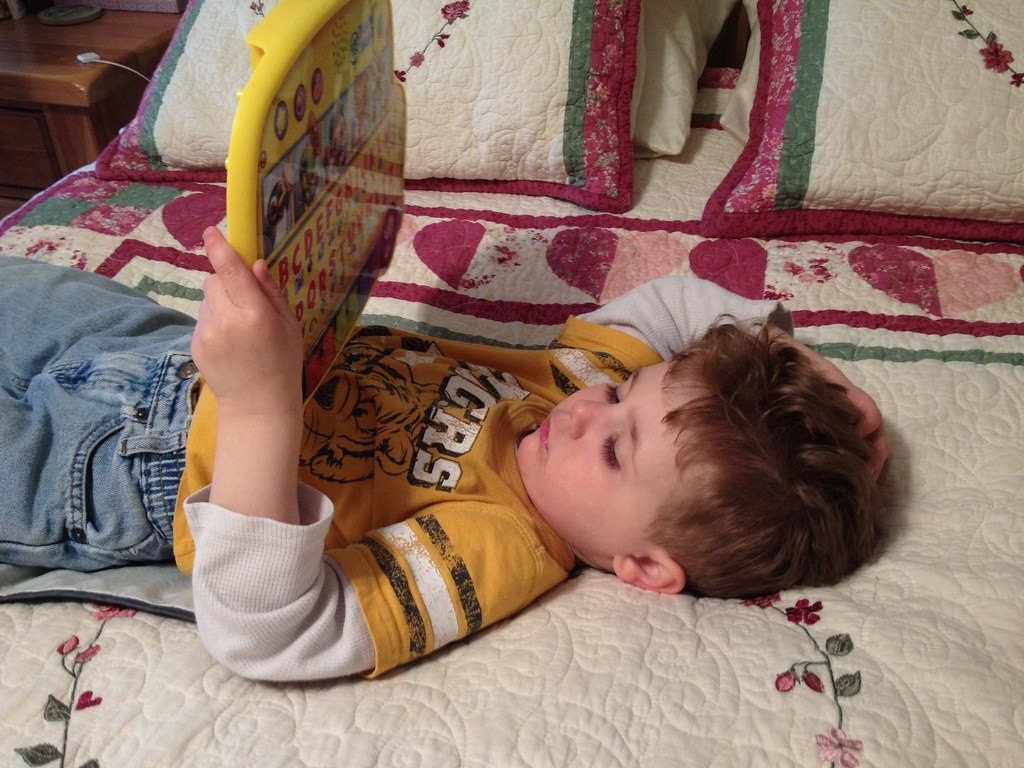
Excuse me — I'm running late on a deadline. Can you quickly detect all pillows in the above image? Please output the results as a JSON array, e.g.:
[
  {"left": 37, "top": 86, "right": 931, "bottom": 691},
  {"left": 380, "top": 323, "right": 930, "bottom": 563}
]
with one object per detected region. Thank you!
[
  {"left": 94, "top": 0, "right": 644, "bottom": 214},
  {"left": 630, "top": 0, "right": 737, "bottom": 162},
  {"left": 701, "top": 0, "right": 1024, "bottom": 245}
]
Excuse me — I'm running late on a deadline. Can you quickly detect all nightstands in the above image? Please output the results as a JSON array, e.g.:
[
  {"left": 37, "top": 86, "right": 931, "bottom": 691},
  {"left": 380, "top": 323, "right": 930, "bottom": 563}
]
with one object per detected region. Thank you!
[{"left": 0, "top": 10, "right": 184, "bottom": 220}]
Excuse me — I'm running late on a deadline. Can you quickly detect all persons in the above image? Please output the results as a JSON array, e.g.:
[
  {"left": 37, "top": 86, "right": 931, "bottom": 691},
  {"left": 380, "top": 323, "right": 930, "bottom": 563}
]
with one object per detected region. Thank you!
[{"left": 0, "top": 225, "right": 892, "bottom": 683}]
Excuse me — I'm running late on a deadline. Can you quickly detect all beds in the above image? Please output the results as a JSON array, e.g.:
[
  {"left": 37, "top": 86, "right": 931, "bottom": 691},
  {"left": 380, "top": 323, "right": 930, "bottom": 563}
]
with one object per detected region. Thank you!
[{"left": 0, "top": 0, "right": 1024, "bottom": 768}]
